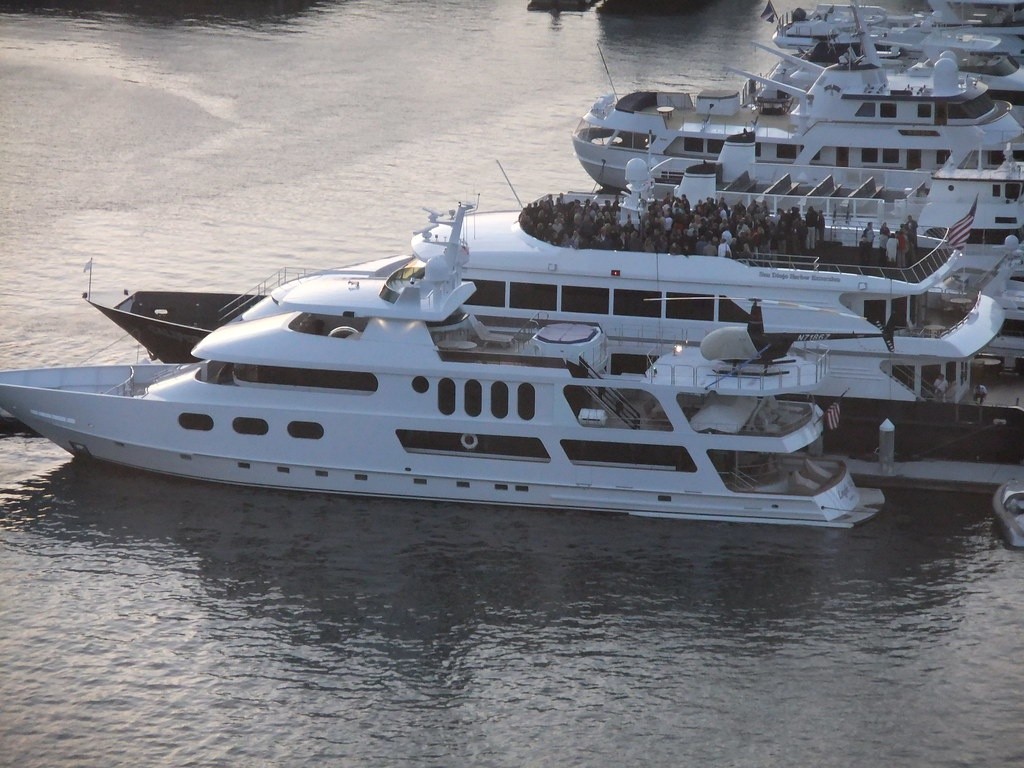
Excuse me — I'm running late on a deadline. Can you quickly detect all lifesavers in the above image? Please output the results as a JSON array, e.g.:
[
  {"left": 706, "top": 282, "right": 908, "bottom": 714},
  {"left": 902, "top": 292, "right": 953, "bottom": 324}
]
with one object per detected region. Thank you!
[{"left": 460, "top": 433, "right": 479, "bottom": 449}]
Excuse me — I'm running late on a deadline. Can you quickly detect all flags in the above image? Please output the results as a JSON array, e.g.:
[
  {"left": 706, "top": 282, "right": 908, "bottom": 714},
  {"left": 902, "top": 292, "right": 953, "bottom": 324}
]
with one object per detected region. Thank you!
[
  {"left": 947, "top": 195, "right": 978, "bottom": 250},
  {"left": 83, "top": 260, "right": 91, "bottom": 272},
  {"left": 760, "top": 1, "right": 775, "bottom": 23},
  {"left": 826, "top": 400, "right": 840, "bottom": 430}
]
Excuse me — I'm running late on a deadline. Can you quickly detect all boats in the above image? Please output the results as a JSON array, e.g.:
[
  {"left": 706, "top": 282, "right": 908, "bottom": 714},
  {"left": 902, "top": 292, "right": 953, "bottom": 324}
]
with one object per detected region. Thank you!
[
  {"left": 84, "top": 0, "right": 1024, "bottom": 503},
  {"left": 0, "top": 202, "right": 893, "bottom": 535}
]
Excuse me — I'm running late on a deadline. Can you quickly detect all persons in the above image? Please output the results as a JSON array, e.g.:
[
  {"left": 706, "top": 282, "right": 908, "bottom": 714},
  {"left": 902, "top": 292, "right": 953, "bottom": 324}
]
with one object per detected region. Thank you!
[
  {"left": 859, "top": 215, "right": 918, "bottom": 266},
  {"left": 934, "top": 374, "right": 948, "bottom": 400},
  {"left": 522, "top": 192, "right": 825, "bottom": 266},
  {"left": 973, "top": 383, "right": 987, "bottom": 405}
]
